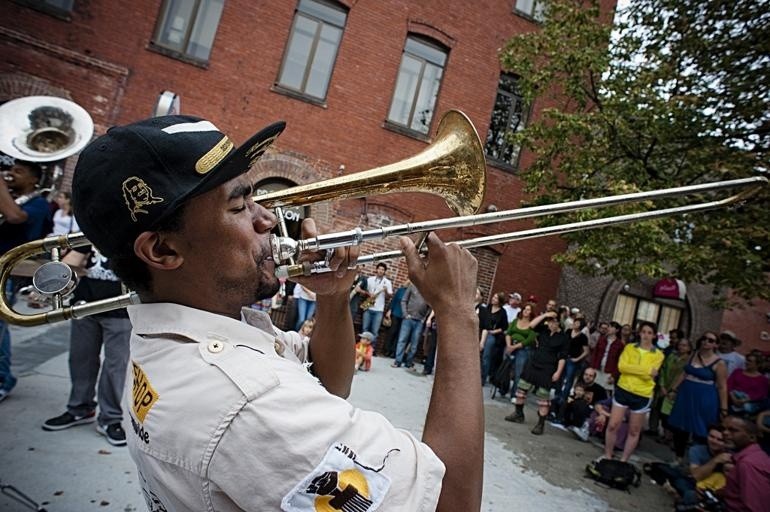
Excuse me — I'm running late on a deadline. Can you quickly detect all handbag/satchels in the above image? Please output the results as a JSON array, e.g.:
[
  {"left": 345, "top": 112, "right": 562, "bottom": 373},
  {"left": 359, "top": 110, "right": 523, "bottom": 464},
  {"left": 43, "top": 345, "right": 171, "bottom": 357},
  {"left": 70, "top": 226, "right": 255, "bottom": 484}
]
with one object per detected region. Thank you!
[{"left": 490, "top": 356, "right": 513, "bottom": 395}]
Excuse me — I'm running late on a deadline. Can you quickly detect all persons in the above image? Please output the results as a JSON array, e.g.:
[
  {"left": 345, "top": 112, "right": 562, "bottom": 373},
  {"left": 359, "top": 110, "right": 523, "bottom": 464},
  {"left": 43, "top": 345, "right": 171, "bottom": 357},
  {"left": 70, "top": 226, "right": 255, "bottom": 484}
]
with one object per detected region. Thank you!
[
  {"left": 74, "top": 114, "right": 484, "bottom": 512},
  {"left": 475, "top": 287, "right": 770, "bottom": 511},
  {"left": 0, "top": 158, "right": 132, "bottom": 446},
  {"left": 251, "top": 275, "right": 316, "bottom": 334},
  {"left": 348, "top": 261, "right": 438, "bottom": 375}
]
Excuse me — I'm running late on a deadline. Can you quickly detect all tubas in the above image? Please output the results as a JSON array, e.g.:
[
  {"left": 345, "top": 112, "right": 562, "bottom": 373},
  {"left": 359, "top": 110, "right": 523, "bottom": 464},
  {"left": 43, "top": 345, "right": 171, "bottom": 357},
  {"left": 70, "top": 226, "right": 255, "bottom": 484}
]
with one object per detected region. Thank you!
[{"left": 0, "top": 95, "right": 94, "bottom": 226}]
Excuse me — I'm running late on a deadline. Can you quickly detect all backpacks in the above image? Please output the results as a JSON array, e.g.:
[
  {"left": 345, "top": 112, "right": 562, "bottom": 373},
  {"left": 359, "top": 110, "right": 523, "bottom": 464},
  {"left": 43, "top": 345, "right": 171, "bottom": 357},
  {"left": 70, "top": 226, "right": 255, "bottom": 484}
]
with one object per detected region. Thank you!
[
  {"left": 585, "top": 458, "right": 641, "bottom": 491},
  {"left": 642, "top": 462, "right": 695, "bottom": 498}
]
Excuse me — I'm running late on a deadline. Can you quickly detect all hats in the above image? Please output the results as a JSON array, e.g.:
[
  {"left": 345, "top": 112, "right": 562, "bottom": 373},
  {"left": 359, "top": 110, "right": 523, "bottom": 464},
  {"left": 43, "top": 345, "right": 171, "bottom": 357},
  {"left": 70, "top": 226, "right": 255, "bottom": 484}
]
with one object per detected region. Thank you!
[
  {"left": 527, "top": 293, "right": 538, "bottom": 302},
  {"left": 71, "top": 115, "right": 286, "bottom": 256},
  {"left": 358, "top": 332, "right": 375, "bottom": 341},
  {"left": 508, "top": 292, "right": 521, "bottom": 301},
  {"left": 720, "top": 330, "right": 737, "bottom": 340}
]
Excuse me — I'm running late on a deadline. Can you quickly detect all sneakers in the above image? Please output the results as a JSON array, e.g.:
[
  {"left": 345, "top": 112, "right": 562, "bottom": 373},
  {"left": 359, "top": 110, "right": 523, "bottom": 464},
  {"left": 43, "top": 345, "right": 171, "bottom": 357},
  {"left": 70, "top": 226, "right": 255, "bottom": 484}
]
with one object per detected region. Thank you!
[
  {"left": 42, "top": 411, "right": 96, "bottom": 431},
  {"left": 505, "top": 412, "right": 526, "bottom": 424},
  {"left": 94, "top": 419, "right": 128, "bottom": 445},
  {"left": 531, "top": 422, "right": 544, "bottom": 434}
]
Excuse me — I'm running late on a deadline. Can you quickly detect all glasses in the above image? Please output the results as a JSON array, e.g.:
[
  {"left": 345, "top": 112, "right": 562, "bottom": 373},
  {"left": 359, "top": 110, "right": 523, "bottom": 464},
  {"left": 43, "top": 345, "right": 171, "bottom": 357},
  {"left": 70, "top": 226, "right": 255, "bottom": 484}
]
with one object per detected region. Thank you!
[{"left": 701, "top": 336, "right": 715, "bottom": 343}]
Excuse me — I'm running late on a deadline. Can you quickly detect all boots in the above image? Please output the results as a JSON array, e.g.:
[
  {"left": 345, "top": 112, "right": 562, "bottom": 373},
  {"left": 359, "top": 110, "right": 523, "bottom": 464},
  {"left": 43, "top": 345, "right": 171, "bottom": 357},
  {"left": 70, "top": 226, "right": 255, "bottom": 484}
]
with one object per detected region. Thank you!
[{"left": 571, "top": 419, "right": 590, "bottom": 442}]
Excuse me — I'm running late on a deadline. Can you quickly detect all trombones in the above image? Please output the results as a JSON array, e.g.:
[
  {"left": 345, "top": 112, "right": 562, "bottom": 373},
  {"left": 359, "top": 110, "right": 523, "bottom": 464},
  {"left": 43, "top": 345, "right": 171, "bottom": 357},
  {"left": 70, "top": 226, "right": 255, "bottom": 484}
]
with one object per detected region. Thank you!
[{"left": 0, "top": 108, "right": 770, "bottom": 325}]
